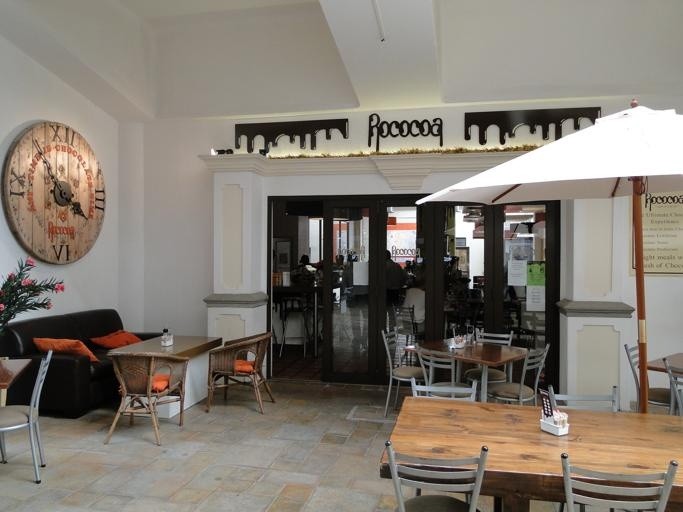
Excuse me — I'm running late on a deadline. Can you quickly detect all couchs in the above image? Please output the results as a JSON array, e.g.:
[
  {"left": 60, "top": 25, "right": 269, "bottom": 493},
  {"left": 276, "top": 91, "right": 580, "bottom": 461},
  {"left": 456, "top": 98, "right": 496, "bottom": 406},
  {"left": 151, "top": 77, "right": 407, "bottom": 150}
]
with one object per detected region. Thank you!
[{"left": 0, "top": 307, "right": 164, "bottom": 420}]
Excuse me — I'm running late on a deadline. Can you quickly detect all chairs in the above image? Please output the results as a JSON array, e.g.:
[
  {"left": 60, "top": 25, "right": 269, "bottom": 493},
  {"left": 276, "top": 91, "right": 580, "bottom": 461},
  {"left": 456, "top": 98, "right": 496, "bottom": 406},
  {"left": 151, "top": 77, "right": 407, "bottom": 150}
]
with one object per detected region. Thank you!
[
  {"left": 408, "top": 373, "right": 479, "bottom": 507},
  {"left": 392, "top": 261, "right": 524, "bottom": 338},
  {"left": 661, "top": 357, "right": 683, "bottom": 415},
  {"left": 279, "top": 295, "right": 309, "bottom": 359},
  {"left": 100, "top": 352, "right": 190, "bottom": 447},
  {"left": 383, "top": 439, "right": 490, "bottom": 512},
  {"left": 560, "top": 452, "right": 679, "bottom": 512},
  {"left": 546, "top": 383, "right": 620, "bottom": 512},
  {"left": 203, "top": 331, "right": 277, "bottom": 416},
  {"left": 0, "top": 348, "right": 53, "bottom": 484},
  {"left": 623, "top": 339, "right": 677, "bottom": 415},
  {"left": 377, "top": 323, "right": 552, "bottom": 419}
]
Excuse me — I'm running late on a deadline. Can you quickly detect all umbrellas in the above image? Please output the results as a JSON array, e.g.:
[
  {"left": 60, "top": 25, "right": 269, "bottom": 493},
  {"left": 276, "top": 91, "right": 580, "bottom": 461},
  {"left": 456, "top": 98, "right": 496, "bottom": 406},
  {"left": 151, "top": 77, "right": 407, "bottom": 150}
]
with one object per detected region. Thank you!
[{"left": 410, "top": 96, "right": 682, "bottom": 414}]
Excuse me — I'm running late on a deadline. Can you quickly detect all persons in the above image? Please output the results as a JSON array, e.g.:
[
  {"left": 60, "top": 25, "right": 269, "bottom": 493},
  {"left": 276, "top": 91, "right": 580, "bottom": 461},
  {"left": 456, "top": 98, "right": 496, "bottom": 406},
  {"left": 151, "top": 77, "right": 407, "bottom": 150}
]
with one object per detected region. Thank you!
[
  {"left": 384, "top": 247, "right": 406, "bottom": 339},
  {"left": 332, "top": 254, "right": 346, "bottom": 280},
  {"left": 298, "top": 254, "right": 315, "bottom": 268}
]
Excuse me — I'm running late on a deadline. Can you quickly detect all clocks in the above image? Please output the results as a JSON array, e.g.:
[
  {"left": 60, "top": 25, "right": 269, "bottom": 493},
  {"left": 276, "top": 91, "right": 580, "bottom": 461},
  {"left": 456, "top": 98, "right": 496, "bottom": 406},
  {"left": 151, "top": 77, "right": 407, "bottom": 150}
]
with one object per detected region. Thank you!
[{"left": 0, "top": 121, "right": 107, "bottom": 266}]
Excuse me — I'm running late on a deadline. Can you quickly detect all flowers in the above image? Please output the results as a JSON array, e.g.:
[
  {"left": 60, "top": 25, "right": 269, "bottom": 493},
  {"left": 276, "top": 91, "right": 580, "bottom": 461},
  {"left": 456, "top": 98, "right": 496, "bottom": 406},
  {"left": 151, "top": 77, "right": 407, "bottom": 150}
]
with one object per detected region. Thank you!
[{"left": 0, "top": 255, "right": 65, "bottom": 336}]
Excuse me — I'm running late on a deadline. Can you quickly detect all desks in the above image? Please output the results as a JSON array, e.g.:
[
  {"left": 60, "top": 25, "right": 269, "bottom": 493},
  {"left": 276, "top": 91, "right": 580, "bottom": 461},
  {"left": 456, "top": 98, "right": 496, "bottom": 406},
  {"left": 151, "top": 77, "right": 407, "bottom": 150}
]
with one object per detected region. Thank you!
[
  {"left": 0, "top": 357, "right": 34, "bottom": 461},
  {"left": 377, "top": 395, "right": 683, "bottom": 512},
  {"left": 638, "top": 351, "right": 683, "bottom": 415}
]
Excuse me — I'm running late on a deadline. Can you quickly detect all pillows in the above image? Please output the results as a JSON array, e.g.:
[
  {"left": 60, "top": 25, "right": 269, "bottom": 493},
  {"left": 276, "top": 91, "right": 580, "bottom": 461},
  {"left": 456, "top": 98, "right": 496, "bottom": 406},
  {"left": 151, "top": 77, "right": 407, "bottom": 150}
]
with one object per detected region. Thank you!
[
  {"left": 89, "top": 330, "right": 145, "bottom": 348},
  {"left": 32, "top": 336, "right": 99, "bottom": 362}
]
